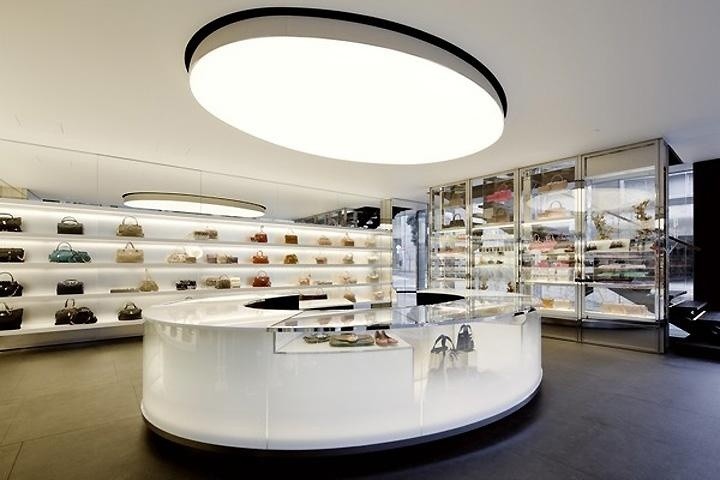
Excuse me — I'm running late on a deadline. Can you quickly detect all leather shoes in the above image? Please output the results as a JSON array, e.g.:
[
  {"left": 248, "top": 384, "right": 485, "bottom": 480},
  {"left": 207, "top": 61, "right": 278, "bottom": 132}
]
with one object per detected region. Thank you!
[
  {"left": 374, "top": 330, "right": 388, "bottom": 346},
  {"left": 381, "top": 329, "right": 398, "bottom": 345}
]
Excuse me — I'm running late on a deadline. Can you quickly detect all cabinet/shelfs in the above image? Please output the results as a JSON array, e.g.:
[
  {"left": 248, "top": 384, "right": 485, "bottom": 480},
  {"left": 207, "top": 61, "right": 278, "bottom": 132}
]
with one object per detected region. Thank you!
[
  {"left": 139, "top": 288, "right": 545, "bottom": 478},
  {"left": 579, "top": 135, "right": 665, "bottom": 355},
  {"left": 430, "top": 180, "right": 470, "bottom": 292},
  {"left": 518, "top": 151, "right": 582, "bottom": 346},
  {"left": 0, "top": 198, "right": 393, "bottom": 354},
  {"left": 300, "top": 207, "right": 360, "bottom": 228},
  {"left": 471, "top": 168, "right": 518, "bottom": 293}
]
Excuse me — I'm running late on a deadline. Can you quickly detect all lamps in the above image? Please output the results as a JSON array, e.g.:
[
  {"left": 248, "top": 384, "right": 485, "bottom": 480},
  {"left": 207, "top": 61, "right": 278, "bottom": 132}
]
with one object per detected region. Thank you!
[
  {"left": 123, "top": 192, "right": 268, "bottom": 220},
  {"left": 187, "top": 13, "right": 506, "bottom": 168}
]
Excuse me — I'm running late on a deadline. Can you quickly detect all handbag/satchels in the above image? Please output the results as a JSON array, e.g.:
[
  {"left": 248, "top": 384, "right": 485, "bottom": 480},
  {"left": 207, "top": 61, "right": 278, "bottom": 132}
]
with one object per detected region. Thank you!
[
  {"left": 450, "top": 193, "right": 464, "bottom": 206},
  {"left": 0, "top": 272, "right": 24, "bottom": 298},
  {"left": 303, "top": 332, "right": 331, "bottom": 344},
  {"left": 542, "top": 233, "right": 557, "bottom": 249},
  {"left": 69, "top": 251, "right": 91, "bottom": 263},
  {"left": 284, "top": 254, "right": 299, "bottom": 264},
  {"left": 56, "top": 278, "right": 84, "bottom": 295},
  {"left": 54, "top": 298, "right": 77, "bottom": 325},
  {"left": 139, "top": 269, "right": 159, "bottom": 292},
  {"left": 366, "top": 271, "right": 379, "bottom": 283},
  {"left": 117, "top": 302, "right": 143, "bottom": 320},
  {"left": 544, "top": 200, "right": 571, "bottom": 218},
  {"left": 254, "top": 232, "right": 268, "bottom": 242},
  {"left": 284, "top": 234, "right": 298, "bottom": 244},
  {"left": 253, "top": 270, "right": 272, "bottom": 288},
  {"left": 0, "top": 247, "right": 25, "bottom": 263},
  {"left": 374, "top": 291, "right": 384, "bottom": 300},
  {"left": 368, "top": 255, "right": 379, "bottom": 265},
  {"left": 547, "top": 174, "right": 568, "bottom": 191},
  {"left": 492, "top": 208, "right": 510, "bottom": 222},
  {"left": 0, "top": 302, "right": 24, "bottom": 331},
  {"left": 342, "top": 271, "right": 358, "bottom": 284},
  {"left": 341, "top": 232, "right": 355, "bottom": 246},
  {"left": 344, "top": 290, "right": 356, "bottom": 301},
  {"left": 493, "top": 184, "right": 512, "bottom": 203},
  {"left": 528, "top": 234, "right": 543, "bottom": 250},
  {"left": 116, "top": 215, "right": 145, "bottom": 238},
  {"left": 445, "top": 353, "right": 481, "bottom": 404},
  {"left": 329, "top": 332, "right": 374, "bottom": 347},
  {"left": 364, "top": 237, "right": 376, "bottom": 248},
  {"left": 167, "top": 246, "right": 189, "bottom": 264},
  {"left": 252, "top": 250, "right": 270, "bottom": 264},
  {"left": 457, "top": 324, "right": 475, "bottom": 352},
  {"left": 57, "top": 216, "right": 84, "bottom": 234},
  {"left": 48, "top": 241, "right": 77, "bottom": 262},
  {"left": 0, "top": 213, "right": 23, "bottom": 232},
  {"left": 315, "top": 256, "right": 328, "bottom": 264},
  {"left": 116, "top": 241, "right": 145, "bottom": 264},
  {"left": 343, "top": 252, "right": 355, "bottom": 264},
  {"left": 299, "top": 275, "right": 310, "bottom": 286},
  {"left": 428, "top": 334, "right": 455, "bottom": 371},
  {"left": 553, "top": 233, "right": 571, "bottom": 250},
  {"left": 70, "top": 306, "right": 98, "bottom": 325},
  {"left": 318, "top": 236, "right": 331, "bottom": 245},
  {"left": 215, "top": 272, "right": 231, "bottom": 290},
  {"left": 206, "top": 253, "right": 218, "bottom": 263}
]
